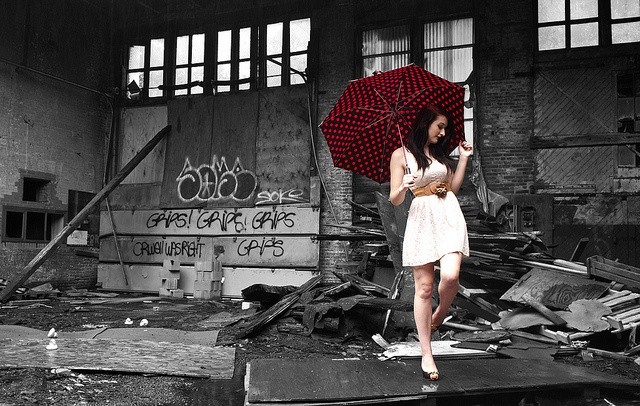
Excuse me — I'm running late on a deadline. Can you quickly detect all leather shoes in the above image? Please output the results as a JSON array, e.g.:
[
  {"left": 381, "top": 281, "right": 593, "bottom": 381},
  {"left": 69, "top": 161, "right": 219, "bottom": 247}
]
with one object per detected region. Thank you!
[
  {"left": 431, "top": 305, "right": 448, "bottom": 335},
  {"left": 420, "top": 361, "right": 440, "bottom": 382}
]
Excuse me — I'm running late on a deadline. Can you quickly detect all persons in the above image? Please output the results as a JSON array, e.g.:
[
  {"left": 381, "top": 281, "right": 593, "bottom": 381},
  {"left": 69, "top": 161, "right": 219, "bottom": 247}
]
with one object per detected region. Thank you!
[{"left": 389, "top": 102, "right": 473, "bottom": 380}]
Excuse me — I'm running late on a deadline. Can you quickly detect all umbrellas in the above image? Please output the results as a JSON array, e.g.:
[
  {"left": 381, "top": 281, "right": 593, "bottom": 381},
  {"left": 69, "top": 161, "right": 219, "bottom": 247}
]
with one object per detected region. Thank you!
[{"left": 318, "top": 62, "right": 466, "bottom": 185}]
